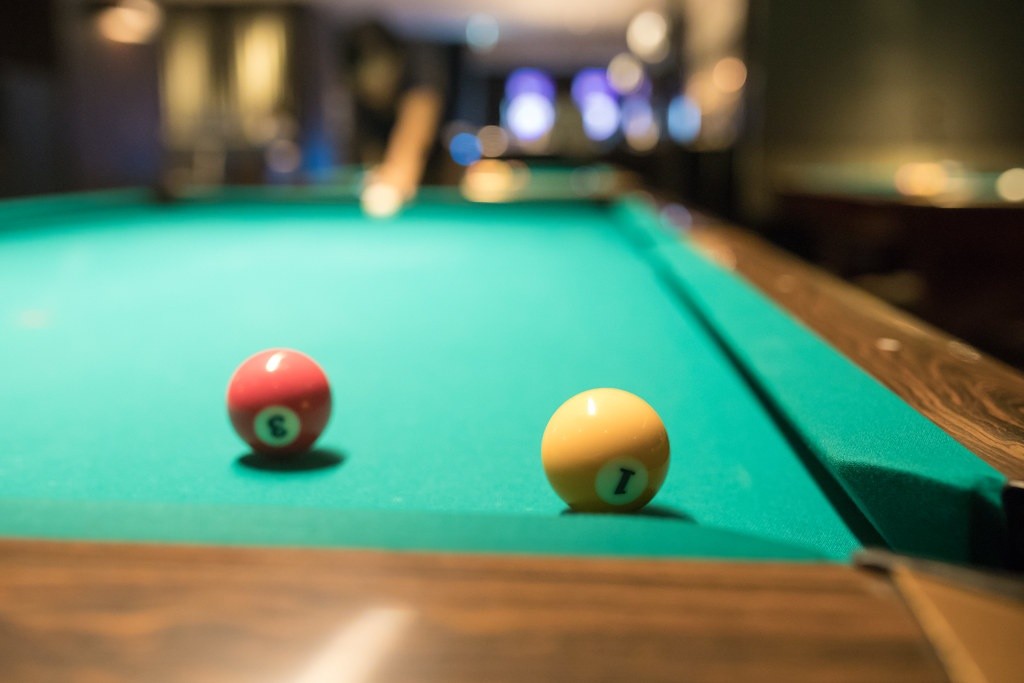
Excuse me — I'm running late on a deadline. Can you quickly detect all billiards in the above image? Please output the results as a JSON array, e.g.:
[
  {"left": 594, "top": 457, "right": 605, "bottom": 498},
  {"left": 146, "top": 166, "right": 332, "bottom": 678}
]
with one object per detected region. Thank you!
[
  {"left": 359, "top": 184, "right": 403, "bottom": 218},
  {"left": 225, "top": 346, "right": 335, "bottom": 457},
  {"left": 540, "top": 385, "right": 672, "bottom": 515}
]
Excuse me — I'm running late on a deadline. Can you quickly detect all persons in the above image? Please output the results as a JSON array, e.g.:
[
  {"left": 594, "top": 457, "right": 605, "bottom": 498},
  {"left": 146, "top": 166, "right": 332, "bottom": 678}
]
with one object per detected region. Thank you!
[{"left": 335, "top": 17, "right": 485, "bottom": 201}]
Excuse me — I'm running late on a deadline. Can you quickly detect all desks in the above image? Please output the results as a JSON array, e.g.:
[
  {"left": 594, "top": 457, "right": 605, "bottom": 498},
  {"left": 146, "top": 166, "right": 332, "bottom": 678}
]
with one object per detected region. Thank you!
[{"left": 1, "top": 166, "right": 1024, "bottom": 683}]
